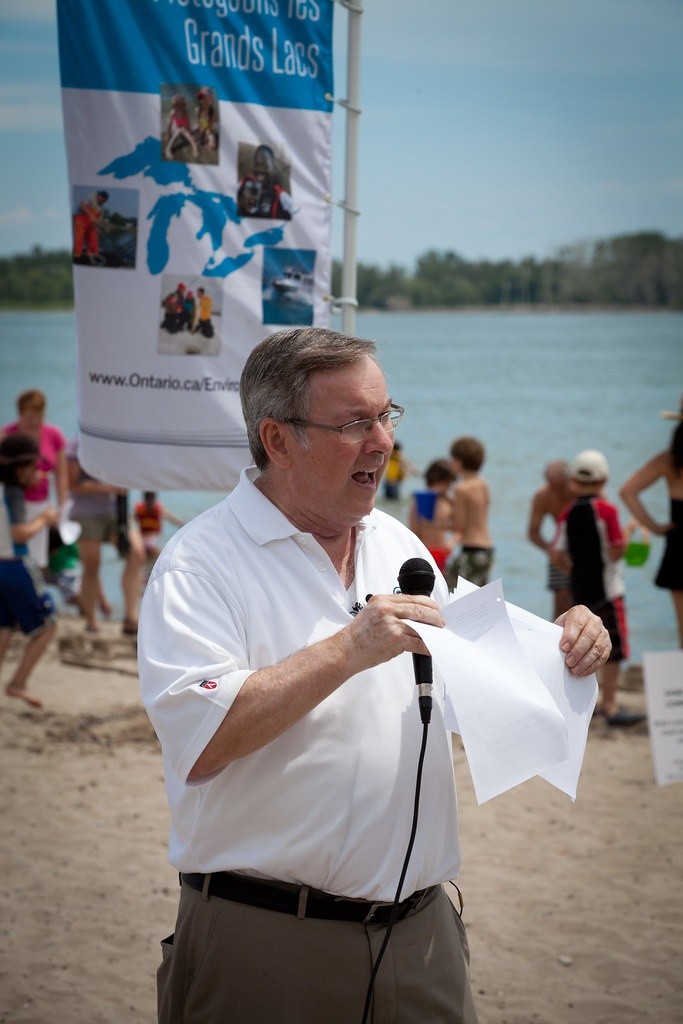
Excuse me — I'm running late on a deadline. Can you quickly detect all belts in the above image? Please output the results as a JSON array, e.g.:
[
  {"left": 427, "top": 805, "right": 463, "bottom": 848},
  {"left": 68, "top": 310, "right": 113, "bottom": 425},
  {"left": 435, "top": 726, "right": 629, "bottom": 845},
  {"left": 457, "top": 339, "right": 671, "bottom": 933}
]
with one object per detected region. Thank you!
[{"left": 183, "top": 872, "right": 440, "bottom": 926}]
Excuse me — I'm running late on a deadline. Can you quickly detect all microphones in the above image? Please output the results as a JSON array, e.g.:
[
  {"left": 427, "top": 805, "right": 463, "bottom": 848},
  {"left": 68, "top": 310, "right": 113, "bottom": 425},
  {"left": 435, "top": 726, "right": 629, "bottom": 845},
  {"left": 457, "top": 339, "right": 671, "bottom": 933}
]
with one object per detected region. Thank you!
[{"left": 397, "top": 558, "right": 435, "bottom": 722}]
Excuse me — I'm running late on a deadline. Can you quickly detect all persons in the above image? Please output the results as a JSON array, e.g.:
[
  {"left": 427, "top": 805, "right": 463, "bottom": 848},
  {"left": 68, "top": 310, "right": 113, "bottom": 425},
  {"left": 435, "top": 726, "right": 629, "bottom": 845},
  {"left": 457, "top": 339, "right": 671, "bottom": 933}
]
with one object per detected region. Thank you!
[
  {"left": 1, "top": 389, "right": 683, "bottom": 1024},
  {"left": 137, "top": 326, "right": 613, "bottom": 1024},
  {"left": 237, "top": 145, "right": 292, "bottom": 219},
  {"left": 72, "top": 190, "right": 109, "bottom": 265},
  {"left": 161, "top": 282, "right": 214, "bottom": 338},
  {"left": 162, "top": 85, "right": 220, "bottom": 161}
]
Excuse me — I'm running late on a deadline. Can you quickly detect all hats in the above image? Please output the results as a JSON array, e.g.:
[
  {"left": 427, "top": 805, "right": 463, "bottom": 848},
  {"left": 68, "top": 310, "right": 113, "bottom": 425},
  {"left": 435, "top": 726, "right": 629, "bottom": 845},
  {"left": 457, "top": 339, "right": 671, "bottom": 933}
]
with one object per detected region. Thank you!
[{"left": 569, "top": 450, "right": 609, "bottom": 482}]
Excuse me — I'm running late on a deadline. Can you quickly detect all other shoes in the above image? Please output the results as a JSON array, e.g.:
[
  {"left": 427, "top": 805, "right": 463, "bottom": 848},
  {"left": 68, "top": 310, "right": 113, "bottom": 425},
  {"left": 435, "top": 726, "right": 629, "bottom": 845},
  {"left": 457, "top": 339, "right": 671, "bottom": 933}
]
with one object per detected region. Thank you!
[{"left": 608, "top": 708, "right": 647, "bottom": 733}]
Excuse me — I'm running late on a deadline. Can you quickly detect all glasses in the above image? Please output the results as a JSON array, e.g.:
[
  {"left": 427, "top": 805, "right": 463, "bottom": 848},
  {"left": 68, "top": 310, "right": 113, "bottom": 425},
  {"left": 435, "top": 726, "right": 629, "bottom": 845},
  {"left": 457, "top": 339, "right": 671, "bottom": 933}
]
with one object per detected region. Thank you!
[{"left": 286, "top": 402, "right": 403, "bottom": 444}]
